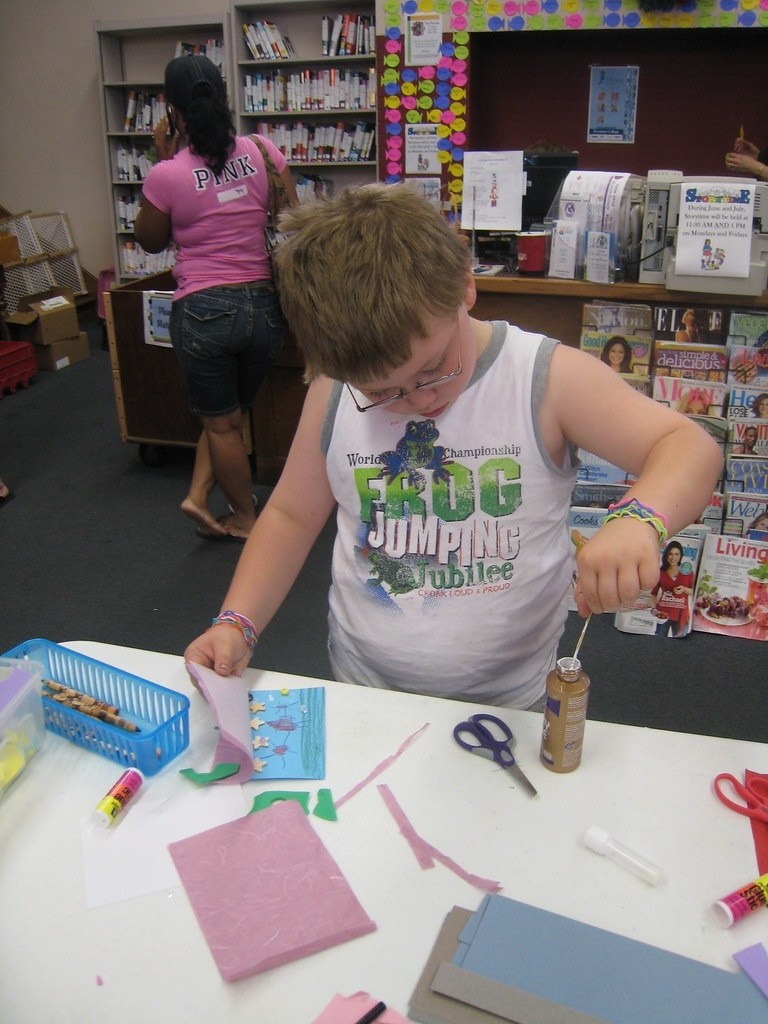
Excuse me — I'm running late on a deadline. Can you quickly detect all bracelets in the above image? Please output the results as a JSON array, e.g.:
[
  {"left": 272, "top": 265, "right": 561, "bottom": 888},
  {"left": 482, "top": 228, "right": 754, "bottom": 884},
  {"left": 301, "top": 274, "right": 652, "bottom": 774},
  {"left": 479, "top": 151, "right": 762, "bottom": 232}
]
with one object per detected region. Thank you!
[
  {"left": 650, "top": 608, "right": 657, "bottom": 613},
  {"left": 756, "top": 165, "right": 765, "bottom": 176},
  {"left": 603, "top": 499, "right": 666, "bottom": 549},
  {"left": 207, "top": 611, "right": 257, "bottom": 649}
]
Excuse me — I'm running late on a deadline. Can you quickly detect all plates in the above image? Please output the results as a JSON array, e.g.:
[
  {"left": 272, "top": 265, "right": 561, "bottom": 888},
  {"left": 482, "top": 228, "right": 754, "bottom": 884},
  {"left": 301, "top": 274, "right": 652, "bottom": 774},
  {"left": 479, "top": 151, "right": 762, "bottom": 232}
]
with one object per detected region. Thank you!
[{"left": 699, "top": 606, "right": 753, "bottom": 626}]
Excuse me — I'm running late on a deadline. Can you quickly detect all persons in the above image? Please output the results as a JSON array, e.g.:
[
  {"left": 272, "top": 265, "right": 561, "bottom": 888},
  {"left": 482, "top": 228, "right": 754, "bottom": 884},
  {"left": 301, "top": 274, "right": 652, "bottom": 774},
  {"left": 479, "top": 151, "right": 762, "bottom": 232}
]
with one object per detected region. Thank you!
[
  {"left": 676, "top": 307, "right": 702, "bottom": 342},
  {"left": 133, "top": 54, "right": 300, "bottom": 543},
  {"left": 185, "top": 184, "right": 722, "bottom": 710},
  {"left": 601, "top": 337, "right": 632, "bottom": 373},
  {"left": 749, "top": 513, "right": 768, "bottom": 532},
  {"left": 675, "top": 389, "right": 715, "bottom": 416},
  {"left": 726, "top": 136, "right": 768, "bottom": 180},
  {"left": 733, "top": 428, "right": 758, "bottom": 454},
  {"left": 750, "top": 393, "right": 768, "bottom": 418},
  {"left": 649, "top": 542, "right": 694, "bottom": 637}
]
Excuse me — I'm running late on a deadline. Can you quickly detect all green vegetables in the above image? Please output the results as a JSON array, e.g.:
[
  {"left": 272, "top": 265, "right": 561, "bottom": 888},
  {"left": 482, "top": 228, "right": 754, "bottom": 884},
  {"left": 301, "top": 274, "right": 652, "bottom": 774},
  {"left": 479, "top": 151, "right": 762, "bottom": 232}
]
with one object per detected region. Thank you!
[
  {"left": 697, "top": 575, "right": 718, "bottom": 598},
  {"left": 747, "top": 563, "right": 768, "bottom": 580}
]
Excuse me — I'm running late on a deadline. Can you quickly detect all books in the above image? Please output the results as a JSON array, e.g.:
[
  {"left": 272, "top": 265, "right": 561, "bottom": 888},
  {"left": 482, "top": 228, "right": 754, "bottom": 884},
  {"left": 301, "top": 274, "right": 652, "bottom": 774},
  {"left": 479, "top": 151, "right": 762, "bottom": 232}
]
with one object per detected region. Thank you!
[
  {"left": 567, "top": 485, "right": 630, "bottom": 610},
  {"left": 566, "top": 299, "right": 768, "bottom": 490},
  {"left": 690, "top": 493, "right": 767, "bottom": 644},
  {"left": 611, "top": 525, "right": 710, "bottom": 638},
  {"left": 116, "top": 12, "right": 378, "bottom": 275}
]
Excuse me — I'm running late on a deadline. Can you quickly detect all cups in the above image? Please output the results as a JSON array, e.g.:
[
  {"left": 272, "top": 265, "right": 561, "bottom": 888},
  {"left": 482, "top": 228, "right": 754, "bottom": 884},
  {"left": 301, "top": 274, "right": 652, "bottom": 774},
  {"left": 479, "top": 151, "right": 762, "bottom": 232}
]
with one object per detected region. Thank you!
[
  {"left": 515, "top": 232, "right": 552, "bottom": 277},
  {"left": 748, "top": 605, "right": 768, "bottom": 630}
]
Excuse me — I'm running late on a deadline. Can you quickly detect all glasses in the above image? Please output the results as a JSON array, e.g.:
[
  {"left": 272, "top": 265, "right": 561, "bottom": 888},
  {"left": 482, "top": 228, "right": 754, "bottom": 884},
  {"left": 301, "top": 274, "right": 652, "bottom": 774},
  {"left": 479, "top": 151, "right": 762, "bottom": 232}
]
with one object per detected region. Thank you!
[{"left": 347, "top": 312, "right": 462, "bottom": 413}]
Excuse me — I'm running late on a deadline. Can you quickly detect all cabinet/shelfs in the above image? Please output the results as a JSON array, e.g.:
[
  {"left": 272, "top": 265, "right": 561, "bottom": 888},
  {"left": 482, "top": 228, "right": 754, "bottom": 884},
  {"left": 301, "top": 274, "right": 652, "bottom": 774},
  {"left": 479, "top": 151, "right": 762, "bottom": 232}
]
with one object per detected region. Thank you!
[{"left": 90, "top": 0, "right": 378, "bottom": 482}]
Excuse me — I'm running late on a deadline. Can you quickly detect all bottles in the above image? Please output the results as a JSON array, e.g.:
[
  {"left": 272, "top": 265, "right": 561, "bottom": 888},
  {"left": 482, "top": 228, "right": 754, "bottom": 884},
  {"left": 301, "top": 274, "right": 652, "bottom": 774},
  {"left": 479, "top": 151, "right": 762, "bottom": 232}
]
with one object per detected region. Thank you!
[{"left": 540, "top": 656, "right": 591, "bottom": 775}]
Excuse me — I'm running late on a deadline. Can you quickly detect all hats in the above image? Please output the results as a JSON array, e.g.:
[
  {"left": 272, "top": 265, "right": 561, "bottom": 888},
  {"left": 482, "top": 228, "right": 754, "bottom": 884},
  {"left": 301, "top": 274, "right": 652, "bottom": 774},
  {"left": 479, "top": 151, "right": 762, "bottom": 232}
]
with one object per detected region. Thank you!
[{"left": 164, "top": 53, "right": 226, "bottom": 107}]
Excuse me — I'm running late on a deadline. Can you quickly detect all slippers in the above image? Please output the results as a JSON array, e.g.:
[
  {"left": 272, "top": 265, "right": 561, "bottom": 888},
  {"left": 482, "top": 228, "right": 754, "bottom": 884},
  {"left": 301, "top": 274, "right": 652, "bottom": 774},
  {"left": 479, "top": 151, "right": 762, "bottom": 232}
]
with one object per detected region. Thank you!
[
  {"left": 0, "top": 493, "right": 13, "bottom": 507},
  {"left": 198, "top": 515, "right": 247, "bottom": 544}
]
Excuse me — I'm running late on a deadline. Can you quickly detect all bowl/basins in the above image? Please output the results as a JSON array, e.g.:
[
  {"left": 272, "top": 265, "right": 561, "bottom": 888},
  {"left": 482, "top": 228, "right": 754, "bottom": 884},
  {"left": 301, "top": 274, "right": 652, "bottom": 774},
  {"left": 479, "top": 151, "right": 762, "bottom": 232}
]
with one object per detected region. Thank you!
[{"left": 655, "top": 612, "right": 669, "bottom": 624}]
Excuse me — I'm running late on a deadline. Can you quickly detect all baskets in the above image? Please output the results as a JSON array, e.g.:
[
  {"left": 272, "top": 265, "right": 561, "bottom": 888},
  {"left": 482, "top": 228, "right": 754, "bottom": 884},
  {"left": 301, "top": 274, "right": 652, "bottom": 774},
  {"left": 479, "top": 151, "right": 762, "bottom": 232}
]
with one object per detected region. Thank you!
[{"left": 1, "top": 638, "right": 192, "bottom": 777}]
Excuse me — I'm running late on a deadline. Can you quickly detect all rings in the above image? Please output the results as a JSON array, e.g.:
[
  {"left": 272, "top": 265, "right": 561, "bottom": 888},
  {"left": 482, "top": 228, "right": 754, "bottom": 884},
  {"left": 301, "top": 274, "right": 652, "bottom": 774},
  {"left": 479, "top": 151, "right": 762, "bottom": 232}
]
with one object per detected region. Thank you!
[{"left": 727, "top": 164, "right": 731, "bottom": 168}]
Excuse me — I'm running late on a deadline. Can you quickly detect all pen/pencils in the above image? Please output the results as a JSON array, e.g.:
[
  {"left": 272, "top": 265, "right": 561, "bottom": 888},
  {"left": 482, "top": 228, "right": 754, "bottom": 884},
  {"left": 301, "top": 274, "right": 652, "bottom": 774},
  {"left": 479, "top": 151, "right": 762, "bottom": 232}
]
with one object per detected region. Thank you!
[{"left": 40, "top": 679, "right": 161, "bottom": 764}]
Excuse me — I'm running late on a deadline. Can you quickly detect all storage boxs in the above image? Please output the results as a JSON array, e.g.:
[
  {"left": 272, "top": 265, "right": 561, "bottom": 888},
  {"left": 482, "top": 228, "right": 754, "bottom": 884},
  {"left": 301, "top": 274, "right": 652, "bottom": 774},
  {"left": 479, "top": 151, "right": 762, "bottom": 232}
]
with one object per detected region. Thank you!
[
  {"left": 4, "top": 286, "right": 79, "bottom": 345},
  {"left": 0, "top": 656, "right": 46, "bottom": 800},
  {"left": 33, "top": 331, "right": 91, "bottom": 372}
]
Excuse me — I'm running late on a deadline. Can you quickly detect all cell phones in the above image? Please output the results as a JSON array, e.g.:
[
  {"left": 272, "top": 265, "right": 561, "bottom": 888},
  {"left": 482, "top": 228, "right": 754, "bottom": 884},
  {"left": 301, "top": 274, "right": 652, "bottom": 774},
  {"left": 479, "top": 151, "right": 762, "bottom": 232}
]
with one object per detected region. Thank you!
[{"left": 166, "top": 105, "right": 176, "bottom": 139}]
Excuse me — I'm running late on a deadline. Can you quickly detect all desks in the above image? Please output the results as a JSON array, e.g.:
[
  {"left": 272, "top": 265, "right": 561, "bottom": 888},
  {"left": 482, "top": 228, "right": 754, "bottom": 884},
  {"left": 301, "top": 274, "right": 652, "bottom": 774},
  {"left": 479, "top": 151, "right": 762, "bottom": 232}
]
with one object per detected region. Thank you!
[{"left": 0, "top": 641, "right": 768, "bottom": 1024}]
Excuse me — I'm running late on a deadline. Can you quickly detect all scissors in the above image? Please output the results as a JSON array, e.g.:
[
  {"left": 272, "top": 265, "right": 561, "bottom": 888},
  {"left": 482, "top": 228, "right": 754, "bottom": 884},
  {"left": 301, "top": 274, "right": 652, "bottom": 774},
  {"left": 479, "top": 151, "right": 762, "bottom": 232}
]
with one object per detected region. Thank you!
[
  {"left": 453, "top": 714, "right": 539, "bottom": 797},
  {"left": 714, "top": 772, "right": 768, "bottom": 823}
]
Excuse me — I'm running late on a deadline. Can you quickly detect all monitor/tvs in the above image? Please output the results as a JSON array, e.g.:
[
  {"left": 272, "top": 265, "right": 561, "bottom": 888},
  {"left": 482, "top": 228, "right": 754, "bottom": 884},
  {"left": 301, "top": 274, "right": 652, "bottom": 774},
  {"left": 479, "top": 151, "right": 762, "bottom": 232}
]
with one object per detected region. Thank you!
[{"left": 468, "top": 153, "right": 580, "bottom": 259}]
